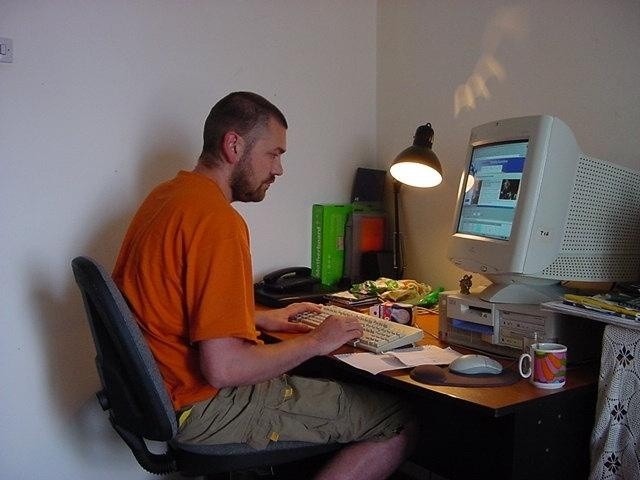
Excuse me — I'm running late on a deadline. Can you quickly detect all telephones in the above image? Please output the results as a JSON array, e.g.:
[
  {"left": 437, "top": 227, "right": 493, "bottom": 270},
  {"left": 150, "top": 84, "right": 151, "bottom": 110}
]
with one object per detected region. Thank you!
[{"left": 259, "top": 267, "right": 320, "bottom": 292}]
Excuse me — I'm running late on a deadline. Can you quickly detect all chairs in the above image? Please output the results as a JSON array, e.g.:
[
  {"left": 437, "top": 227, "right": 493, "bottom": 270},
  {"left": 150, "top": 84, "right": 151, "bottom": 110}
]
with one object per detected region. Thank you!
[{"left": 71, "top": 255, "right": 347, "bottom": 480}]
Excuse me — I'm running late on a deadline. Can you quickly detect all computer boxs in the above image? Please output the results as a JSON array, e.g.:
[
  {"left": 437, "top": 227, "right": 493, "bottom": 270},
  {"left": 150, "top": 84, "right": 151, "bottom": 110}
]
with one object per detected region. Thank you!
[{"left": 438, "top": 285, "right": 561, "bottom": 359}]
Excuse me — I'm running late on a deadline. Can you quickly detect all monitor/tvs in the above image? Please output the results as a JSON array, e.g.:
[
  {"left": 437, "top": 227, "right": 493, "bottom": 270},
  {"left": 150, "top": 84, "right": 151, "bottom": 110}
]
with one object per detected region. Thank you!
[{"left": 446, "top": 114, "right": 640, "bottom": 305}]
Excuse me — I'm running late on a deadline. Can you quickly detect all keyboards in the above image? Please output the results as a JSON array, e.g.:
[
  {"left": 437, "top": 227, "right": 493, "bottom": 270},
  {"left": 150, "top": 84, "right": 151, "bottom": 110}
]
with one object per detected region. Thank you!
[{"left": 289, "top": 304, "right": 423, "bottom": 354}]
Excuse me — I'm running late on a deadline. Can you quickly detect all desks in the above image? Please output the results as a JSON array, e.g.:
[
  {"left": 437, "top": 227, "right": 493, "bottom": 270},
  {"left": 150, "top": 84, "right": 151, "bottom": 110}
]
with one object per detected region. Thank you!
[{"left": 256, "top": 305, "right": 601, "bottom": 479}]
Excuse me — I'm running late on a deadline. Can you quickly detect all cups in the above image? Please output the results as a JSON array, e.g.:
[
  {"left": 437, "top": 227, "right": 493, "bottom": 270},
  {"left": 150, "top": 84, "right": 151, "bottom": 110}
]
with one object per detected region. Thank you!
[{"left": 516, "top": 342, "right": 569, "bottom": 390}]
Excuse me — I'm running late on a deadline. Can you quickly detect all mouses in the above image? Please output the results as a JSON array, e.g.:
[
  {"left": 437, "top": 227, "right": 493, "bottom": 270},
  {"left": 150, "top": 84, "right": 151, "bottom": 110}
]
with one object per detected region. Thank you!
[{"left": 448, "top": 353, "right": 503, "bottom": 376}]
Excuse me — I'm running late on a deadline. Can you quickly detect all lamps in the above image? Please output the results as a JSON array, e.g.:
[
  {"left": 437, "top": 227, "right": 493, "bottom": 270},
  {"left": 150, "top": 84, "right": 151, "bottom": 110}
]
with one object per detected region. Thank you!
[{"left": 388, "top": 122, "right": 444, "bottom": 281}]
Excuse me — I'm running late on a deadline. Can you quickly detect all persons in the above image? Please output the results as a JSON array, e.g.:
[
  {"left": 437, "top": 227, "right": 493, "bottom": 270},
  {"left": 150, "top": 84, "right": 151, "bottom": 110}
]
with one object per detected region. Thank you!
[{"left": 110, "top": 91, "right": 423, "bottom": 480}]
